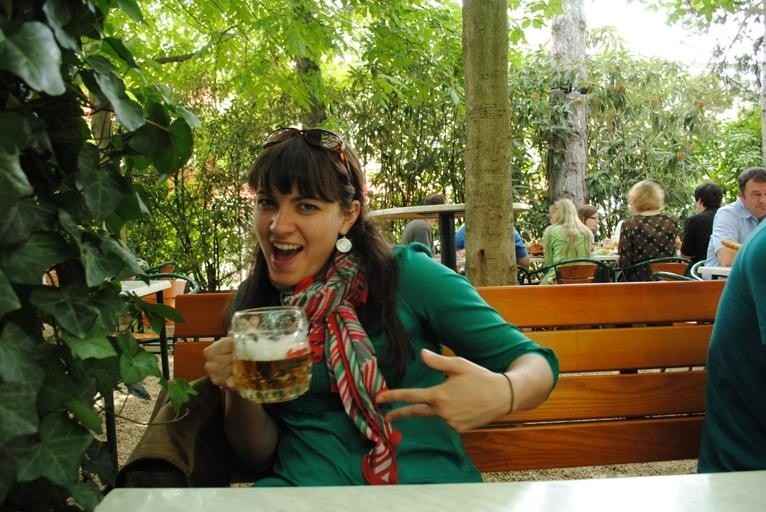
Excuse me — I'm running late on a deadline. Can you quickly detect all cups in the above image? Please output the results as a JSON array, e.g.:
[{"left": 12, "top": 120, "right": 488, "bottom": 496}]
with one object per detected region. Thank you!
[{"left": 230, "top": 305, "right": 314, "bottom": 405}]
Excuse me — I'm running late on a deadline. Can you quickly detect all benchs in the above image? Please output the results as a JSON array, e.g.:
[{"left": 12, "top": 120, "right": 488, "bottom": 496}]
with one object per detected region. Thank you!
[{"left": 171, "top": 278, "right": 728, "bottom": 482}]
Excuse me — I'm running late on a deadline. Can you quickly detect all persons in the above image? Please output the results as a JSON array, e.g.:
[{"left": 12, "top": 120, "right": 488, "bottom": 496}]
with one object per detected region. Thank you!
[
  {"left": 397, "top": 193, "right": 599, "bottom": 285},
  {"left": 202, "top": 128, "right": 559, "bottom": 487},
  {"left": 615, "top": 167, "right": 766, "bottom": 281},
  {"left": 697, "top": 218, "right": 766, "bottom": 473}
]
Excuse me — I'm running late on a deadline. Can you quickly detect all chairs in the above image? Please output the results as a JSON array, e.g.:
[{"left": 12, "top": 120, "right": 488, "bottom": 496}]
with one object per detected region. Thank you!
[
  {"left": 519, "top": 250, "right": 708, "bottom": 284},
  {"left": 43, "top": 262, "right": 204, "bottom": 352}
]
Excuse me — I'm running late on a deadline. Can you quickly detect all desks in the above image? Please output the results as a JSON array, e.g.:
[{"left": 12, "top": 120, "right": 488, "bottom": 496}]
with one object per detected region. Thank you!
[
  {"left": 93, "top": 469, "right": 766, "bottom": 512},
  {"left": 364, "top": 201, "right": 532, "bottom": 272},
  {"left": 115, "top": 278, "right": 172, "bottom": 380}
]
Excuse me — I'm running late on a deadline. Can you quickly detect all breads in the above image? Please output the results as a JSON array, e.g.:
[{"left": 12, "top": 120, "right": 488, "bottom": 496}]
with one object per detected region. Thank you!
[{"left": 720, "top": 240, "right": 740, "bottom": 251}]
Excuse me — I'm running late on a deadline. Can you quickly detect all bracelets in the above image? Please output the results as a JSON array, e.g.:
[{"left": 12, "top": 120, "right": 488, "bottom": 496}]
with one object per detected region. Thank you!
[{"left": 502, "top": 373, "right": 514, "bottom": 415}]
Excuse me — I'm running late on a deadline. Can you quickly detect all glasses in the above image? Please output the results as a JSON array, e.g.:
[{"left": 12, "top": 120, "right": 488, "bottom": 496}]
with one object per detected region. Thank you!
[{"left": 262, "top": 128, "right": 353, "bottom": 185}]
